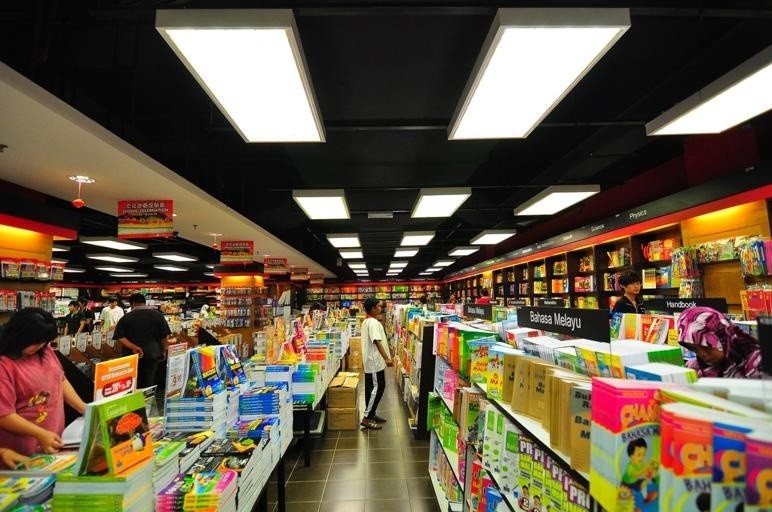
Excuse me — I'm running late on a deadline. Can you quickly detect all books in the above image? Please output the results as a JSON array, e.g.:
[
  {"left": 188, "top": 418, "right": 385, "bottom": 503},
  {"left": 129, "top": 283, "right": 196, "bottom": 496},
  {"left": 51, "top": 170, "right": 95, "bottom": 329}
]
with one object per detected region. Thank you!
[
  {"left": 606, "top": 248, "right": 630, "bottom": 269},
  {"left": 641, "top": 265, "right": 679, "bottom": 289},
  {"left": 249, "top": 305, "right": 353, "bottom": 403},
  {"left": 216, "top": 344, "right": 230, "bottom": 386},
  {"left": 0, "top": 288, "right": 56, "bottom": 312},
  {"left": 190, "top": 347, "right": 223, "bottom": 397},
  {"left": 180, "top": 344, "right": 206, "bottom": 397},
  {"left": 609, "top": 367, "right": 771, "bottom": 511},
  {"left": 1, "top": 471, "right": 57, "bottom": 511},
  {"left": 608, "top": 313, "right": 686, "bottom": 367},
  {"left": 97, "top": 391, "right": 154, "bottom": 476},
  {"left": 33, "top": 450, "right": 80, "bottom": 471},
  {"left": 641, "top": 238, "right": 680, "bottom": 262},
  {"left": 642, "top": 294, "right": 664, "bottom": 300},
  {"left": 385, "top": 302, "right": 462, "bottom": 430},
  {"left": 73, "top": 393, "right": 127, "bottom": 478},
  {"left": 220, "top": 345, "right": 248, "bottom": 386},
  {"left": 153, "top": 387, "right": 294, "bottom": 512},
  {"left": 608, "top": 296, "right": 621, "bottom": 315},
  {"left": 427, "top": 319, "right": 608, "bottom": 512},
  {"left": 53, "top": 467, "right": 154, "bottom": 512},
  {"left": 60, "top": 417, "right": 85, "bottom": 449},
  {"left": 0, "top": 256, "right": 65, "bottom": 281},
  {"left": 603, "top": 272, "right": 621, "bottom": 292}
]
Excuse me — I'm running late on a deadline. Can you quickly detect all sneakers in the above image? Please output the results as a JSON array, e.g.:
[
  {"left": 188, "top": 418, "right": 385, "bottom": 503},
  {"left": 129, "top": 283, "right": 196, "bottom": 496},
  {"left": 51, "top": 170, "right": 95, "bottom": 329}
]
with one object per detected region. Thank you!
[
  {"left": 360, "top": 417, "right": 382, "bottom": 429},
  {"left": 372, "top": 415, "right": 386, "bottom": 423}
]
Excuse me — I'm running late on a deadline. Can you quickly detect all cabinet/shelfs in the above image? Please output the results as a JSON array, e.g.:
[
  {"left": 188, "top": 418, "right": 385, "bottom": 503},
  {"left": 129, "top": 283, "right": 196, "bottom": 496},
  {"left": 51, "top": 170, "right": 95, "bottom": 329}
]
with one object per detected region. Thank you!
[
  {"left": 386, "top": 309, "right": 427, "bottom": 433},
  {"left": 691, "top": 251, "right": 772, "bottom": 317},
  {"left": 0, "top": 275, "right": 58, "bottom": 315},
  {"left": 49, "top": 284, "right": 221, "bottom": 311},
  {"left": 305, "top": 286, "right": 442, "bottom": 308},
  {"left": 494, "top": 220, "right": 685, "bottom": 308},
  {"left": 254, "top": 293, "right": 271, "bottom": 328},
  {"left": 425, "top": 353, "right": 609, "bottom": 512},
  {"left": 443, "top": 274, "right": 484, "bottom": 301},
  {"left": 220, "top": 293, "right": 251, "bottom": 328}
]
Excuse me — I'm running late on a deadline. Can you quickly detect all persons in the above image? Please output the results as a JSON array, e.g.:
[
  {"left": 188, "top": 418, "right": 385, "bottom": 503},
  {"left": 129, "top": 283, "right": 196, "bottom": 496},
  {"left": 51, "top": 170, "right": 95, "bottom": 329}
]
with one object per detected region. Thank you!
[
  {"left": 112, "top": 294, "right": 173, "bottom": 389},
  {"left": 98, "top": 296, "right": 125, "bottom": 333},
  {"left": 207, "top": 306, "right": 216, "bottom": 318},
  {"left": 76, "top": 298, "right": 95, "bottom": 335},
  {"left": 0, "top": 447, "right": 33, "bottom": 471},
  {"left": 1, "top": 307, "right": 89, "bottom": 469},
  {"left": 475, "top": 288, "right": 490, "bottom": 305},
  {"left": 64, "top": 300, "right": 87, "bottom": 337},
  {"left": 199, "top": 304, "right": 209, "bottom": 318},
  {"left": 676, "top": 306, "right": 770, "bottom": 379},
  {"left": 359, "top": 298, "right": 396, "bottom": 430},
  {"left": 611, "top": 270, "right": 646, "bottom": 318}
]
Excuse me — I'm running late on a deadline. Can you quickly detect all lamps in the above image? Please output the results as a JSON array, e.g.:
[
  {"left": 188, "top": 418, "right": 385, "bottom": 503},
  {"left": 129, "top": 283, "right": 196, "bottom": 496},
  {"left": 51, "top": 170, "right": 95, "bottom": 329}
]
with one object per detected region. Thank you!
[
  {"left": 293, "top": 189, "right": 351, "bottom": 221},
  {"left": 399, "top": 231, "right": 436, "bottom": 247},
  {"left": 385, "top": 272, "right": 399, "bottom": 276},
  {"left": 418, "top": 271, "right": 433, "bottom": 276},
  {"left": 347, "top": 261, "right": 366, "bottom": 269},
  {"left": 155, "top": 8, "right": 327, "bottom": 145},
  {"left": 644, "top": 44, "right": 772, "bottom": 140},
  {"left": 425, "top": 267, "right": 443, "bottom": 272},
  {"left": 389, "top": 261, "right": 408, "bottom": 269},
  {"left": 327, "top": 233, "right": 362, "bottom": 249},
  {"left": 352, "top": 269, "right": 368, "bottom": 274},
  {"left": 513, "top": 185, "right": 602, "bottom": 220},
  {"left": 356, "top": 274, "right": 369, "bottom": 277},
  {"left": 393, "top": 248, "right": 419, "bottom": 258},
  {"left": 432, "top": 260, "right": 456, "bottom": 267},
  {"left": 443, "top": 5, "right": 631, "bottom": 145},
  {"left": 470, "top": 230, "right": 518, "bottom": 246},
  {"left": 339, "top": 249, "right": 363, "bottom": 258},
  {"left": 387, "top": 269, "right": 402, "bottom": 273},
  {"left": 447, "top": 246, "right": 480, "bottom": 257},
  {"left": 410, "top": 187, "right": 471, "bottom": 219}
]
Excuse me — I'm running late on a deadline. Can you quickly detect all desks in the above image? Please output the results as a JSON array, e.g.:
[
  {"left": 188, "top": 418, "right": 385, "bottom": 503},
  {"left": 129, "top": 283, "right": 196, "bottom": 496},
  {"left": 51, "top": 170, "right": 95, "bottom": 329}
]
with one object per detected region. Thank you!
[
  {"left": 245, "top": 438, "right": 300, "bottom": 512},
  {"left": 291, "top": 360, "right": 345, "bottom": 467}
]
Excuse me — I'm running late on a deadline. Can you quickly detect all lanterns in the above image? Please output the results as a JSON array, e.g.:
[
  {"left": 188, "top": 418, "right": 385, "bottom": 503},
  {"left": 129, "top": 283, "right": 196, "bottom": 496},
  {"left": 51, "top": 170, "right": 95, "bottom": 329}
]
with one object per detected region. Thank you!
[
  {"left": 213, "top": 244, "right": 218, "bottom": 248},
  {"left": 72, "top": 199, "right": 85, "bottom": 209}
]
